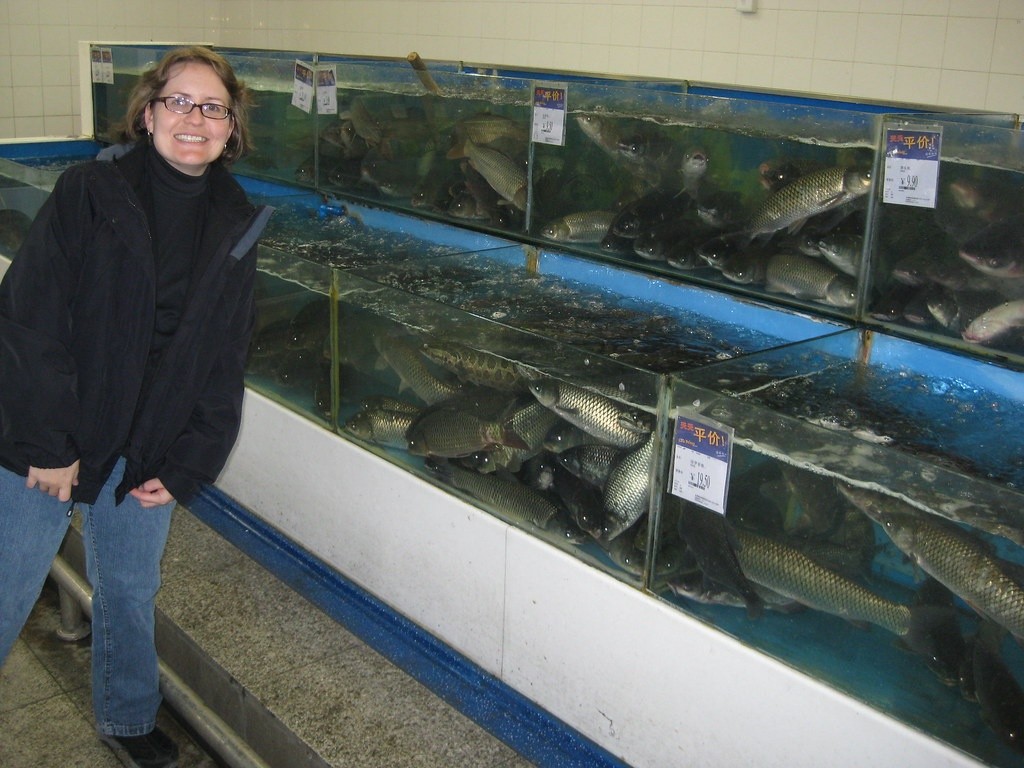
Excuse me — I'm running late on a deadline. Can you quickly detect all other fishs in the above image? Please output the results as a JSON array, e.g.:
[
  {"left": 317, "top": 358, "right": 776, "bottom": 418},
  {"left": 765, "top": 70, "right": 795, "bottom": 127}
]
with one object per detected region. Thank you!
[{"left": 244, "top": 89, "right": 1024, "bottom": 746}]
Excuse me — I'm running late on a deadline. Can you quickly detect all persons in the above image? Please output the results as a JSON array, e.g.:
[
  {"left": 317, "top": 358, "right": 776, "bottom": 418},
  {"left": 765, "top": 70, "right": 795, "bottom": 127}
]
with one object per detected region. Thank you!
[{"left": 0, "top": 44, "right": 258, "bottom": 768}]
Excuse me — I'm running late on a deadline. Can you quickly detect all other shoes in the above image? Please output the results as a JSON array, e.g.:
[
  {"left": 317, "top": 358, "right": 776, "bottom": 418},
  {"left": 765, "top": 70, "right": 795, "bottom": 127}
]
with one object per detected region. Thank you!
[{"left": 100, "top": 727, "right": 182, "bottom": 768}]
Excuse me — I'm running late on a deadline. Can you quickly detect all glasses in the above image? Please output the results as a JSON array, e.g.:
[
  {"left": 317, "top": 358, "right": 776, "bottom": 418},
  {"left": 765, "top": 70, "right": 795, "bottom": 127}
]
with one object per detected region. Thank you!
[{"left": 151, "top": 96, "right": 233, "bottom": 120}]
